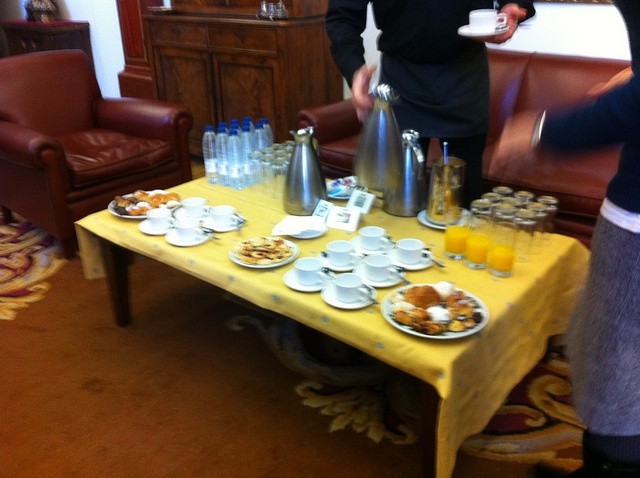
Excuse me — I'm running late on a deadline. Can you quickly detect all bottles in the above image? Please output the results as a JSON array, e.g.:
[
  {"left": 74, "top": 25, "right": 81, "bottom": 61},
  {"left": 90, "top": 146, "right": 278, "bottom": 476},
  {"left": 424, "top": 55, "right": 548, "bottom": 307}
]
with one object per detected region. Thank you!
[
  {"left": 256, "top": 1, "right": 290, "bottom": 20},
  {"left": 202, "top": 119, "right": 275, "bottom": 190}
]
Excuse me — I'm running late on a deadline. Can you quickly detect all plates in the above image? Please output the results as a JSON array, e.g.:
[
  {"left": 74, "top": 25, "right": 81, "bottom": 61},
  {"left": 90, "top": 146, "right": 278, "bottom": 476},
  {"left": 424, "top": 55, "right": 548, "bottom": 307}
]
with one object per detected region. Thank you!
[
  {"left": 108, "top": 188, "right": 182, "bottom": 219},
  {"left": 317, "top": 249, "right": 365, "bottom": 272},
  {"left": 325, "top": 179, "right": 368, "bottom": 200},
  {"left": 457, "top": 24, "right": 509, "bottom": 37},
  {"left": 387, "top": 245, "right": 434, "bottom": 271},
  {"left": 138, "top": 219, "right": 170, "bottom": 236},
  {"left": 352, "top": 264, "right": 404, "bottom": 288},
  {"left": 416, "top": 206, "right": 477, "bottom": 230},
  {"left": 172, "top": 205, "right": 213, "bottom": 221},
  {"left": 291, "top": 232, "right": 324, "bottom": 240},
  {"left": 381, "top": 283, "right": 489, "bottom": 340},
  {"left": 282, "top": 268, "right": 335, "bottom": 293},
  {"left": 320, "top": 283, "right": 377, "bottom": 311},
  {"left": 164, "top": 228, "right": 212, "bottom": 247},
  {"left": 201, "top": 214, "right": 244, "bottom": 232},
  {"left": 351, "top": 235, "right": 395, "bottom": 256},
  {"left": 228, "top": 237, "right": 300, "bottom": 269}
]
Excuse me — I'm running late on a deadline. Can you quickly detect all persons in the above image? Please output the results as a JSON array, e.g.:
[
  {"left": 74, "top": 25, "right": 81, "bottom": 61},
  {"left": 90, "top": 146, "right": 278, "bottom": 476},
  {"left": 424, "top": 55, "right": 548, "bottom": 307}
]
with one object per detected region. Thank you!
[
  {"left": 485, "top": 0, "right": 640, "bottom": 475},
  {"left": 326, "top": 0, "right": 534, "bottom": 211}
]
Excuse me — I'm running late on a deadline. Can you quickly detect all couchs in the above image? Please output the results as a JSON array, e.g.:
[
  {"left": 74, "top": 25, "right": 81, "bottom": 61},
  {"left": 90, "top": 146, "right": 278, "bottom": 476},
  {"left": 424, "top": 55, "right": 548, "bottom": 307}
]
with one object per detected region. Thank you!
[
  {"left": 1, "top": 47, "right": 196, "bottom": 244},
  {"left": 296, "top": 48, "right": 631, "bottom": 250}
]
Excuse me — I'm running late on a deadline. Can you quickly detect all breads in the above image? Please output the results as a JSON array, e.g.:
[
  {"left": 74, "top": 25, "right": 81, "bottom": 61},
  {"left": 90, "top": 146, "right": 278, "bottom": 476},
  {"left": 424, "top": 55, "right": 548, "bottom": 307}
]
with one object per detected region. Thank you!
[
  {"left": 115, "top": 191, "right": 181, "bottom": 216},
  {"left": 393, "top": 282, "right": 477, "bottom": 334}
]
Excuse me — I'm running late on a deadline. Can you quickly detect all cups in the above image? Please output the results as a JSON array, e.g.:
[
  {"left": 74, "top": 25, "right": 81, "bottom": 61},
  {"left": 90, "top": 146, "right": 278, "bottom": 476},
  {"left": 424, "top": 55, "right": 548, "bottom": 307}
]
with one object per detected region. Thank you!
[
  {"left": 490, "top": 203, "right": 513, "bottom": 242},
  {"left": 146, "top": 208, "right": 176, "bottom": 229},
  {"left": 210, "top": 205, "right": 237, "bottom": 226},
  {"left": 443, "top": 207, "right": 471, "bottom": 261},
  {"left": 364, "top": 253, "right": 398, "bottom": 283},
  {"left": 465, "top": 214, "right": 494, "bottom": 269},
  {"left": 501, "top": 196, "right": 531, "bottom": 207},
  {"left": 513, "top": 190, "right": 536, "bottom": 201},
  {"left": 359, "top": 226, "right": 389, "bottom": 251},
  {"left": 492, "top": 185, "right": 514, "bottom": 197},
  {"left": 248, "top": 140, "right": 294, "bottom": 199},
  {"left": 181, "top": 197, "right": 210, "bottom": 216},
  {"left": 488, "top": 221, "right": 519, "bottom": 278},
  {"left": 468, "top": 8, "right": 506, "bottom": 32},
  {"left": 326, "top": 240, "right": 358, "bottom": 266},
  {"left": 293, "top": 257, "right": 328, "bottom": 284},
  {"left": 332, "top": 273, "right": 364, "bottom": 302},
  {"left": 526, "top": 201, "right": 545, "bottom": 255},
  {"left": 481, "top": 192, "right": 501, "bottom": 204},
  {"left": 469, "top": 199, "right": 492, "bottom": 229},
  {"left": 535, "top": 195, "right": 559, "bottom": 240},
  {"left": 426, "top": 156, "right": 465, "bottom": 225},
  {"left": 512, "top": 208, "right": 533, "bottom": 265},
  {"left": 173, "top": 218, "right": 203, "bottom": 240},
  {"left": 397, "top": 238, "right": 432, "bottom": 266}
]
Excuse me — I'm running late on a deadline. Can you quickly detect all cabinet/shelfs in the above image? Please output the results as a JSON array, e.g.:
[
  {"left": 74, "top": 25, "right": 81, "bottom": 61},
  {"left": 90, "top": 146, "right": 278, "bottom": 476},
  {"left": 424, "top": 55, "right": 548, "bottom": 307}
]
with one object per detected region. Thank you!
[
  {"left": 140, "top": 7, "right": 346, "bottom": 159},
  {"left": 0, "top": 19, "right": 95, "bottom": 71}
]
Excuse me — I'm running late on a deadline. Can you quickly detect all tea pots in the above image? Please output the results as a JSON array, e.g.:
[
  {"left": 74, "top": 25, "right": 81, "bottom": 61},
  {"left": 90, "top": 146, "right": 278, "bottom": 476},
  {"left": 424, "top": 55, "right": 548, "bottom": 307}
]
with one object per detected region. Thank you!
[
  {"left": 351, "top": 81, "right": 404, "bottom": 192},
  {"left": 382, "top": 128, "right": 428, "bottom": 218},
  {"left": 282, "top": 124, "right": 328, "bottom": 216}
]
